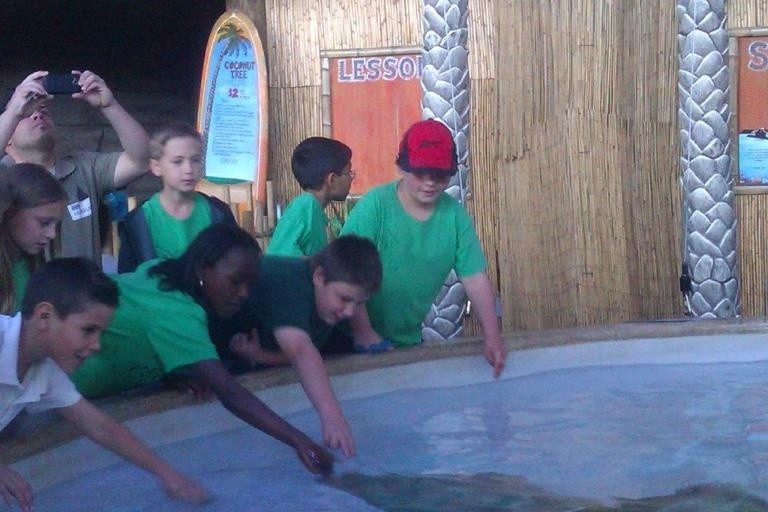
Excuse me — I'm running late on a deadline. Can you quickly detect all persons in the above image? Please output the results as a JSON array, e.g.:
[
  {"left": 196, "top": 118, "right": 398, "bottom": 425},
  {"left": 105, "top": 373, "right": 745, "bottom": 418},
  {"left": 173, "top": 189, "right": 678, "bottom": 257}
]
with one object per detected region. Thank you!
[
  {"left": 68, "top": 223, "right": 334, "bottom": 483},
  {"left": 339, "top": 119, "right": 510, "bottom": 382},
  {"left": 227, "top": 237, "right": 382, "bottom": 457},
  {"left": 1, "top": 258, "right": 221, "bottom": 510},
  {"left": 0, "top": 161, "right": 68, "bottom": 327},
  {"left": 262, "top": 138, "right": 355, "bottom": 262},
  {"left": 0, "top": 65, "right": 149, "bottom": 276},
  {"left": 115, "top": 124, "right": 237, "bottom": 275}
]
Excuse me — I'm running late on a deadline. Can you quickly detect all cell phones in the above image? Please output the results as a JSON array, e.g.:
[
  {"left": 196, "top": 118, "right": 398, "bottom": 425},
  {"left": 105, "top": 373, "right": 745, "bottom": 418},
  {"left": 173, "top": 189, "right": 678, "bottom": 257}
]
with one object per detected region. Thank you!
[{"left": 42, "top": 74, "right": 81, "bottom": 95}]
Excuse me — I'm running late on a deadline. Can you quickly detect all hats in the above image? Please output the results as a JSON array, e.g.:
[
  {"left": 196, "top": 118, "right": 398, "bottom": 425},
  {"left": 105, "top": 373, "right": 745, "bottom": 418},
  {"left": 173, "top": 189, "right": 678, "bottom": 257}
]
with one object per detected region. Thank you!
[{"left": 399, "top": 117, "right": 458, "bottom": 176}]
